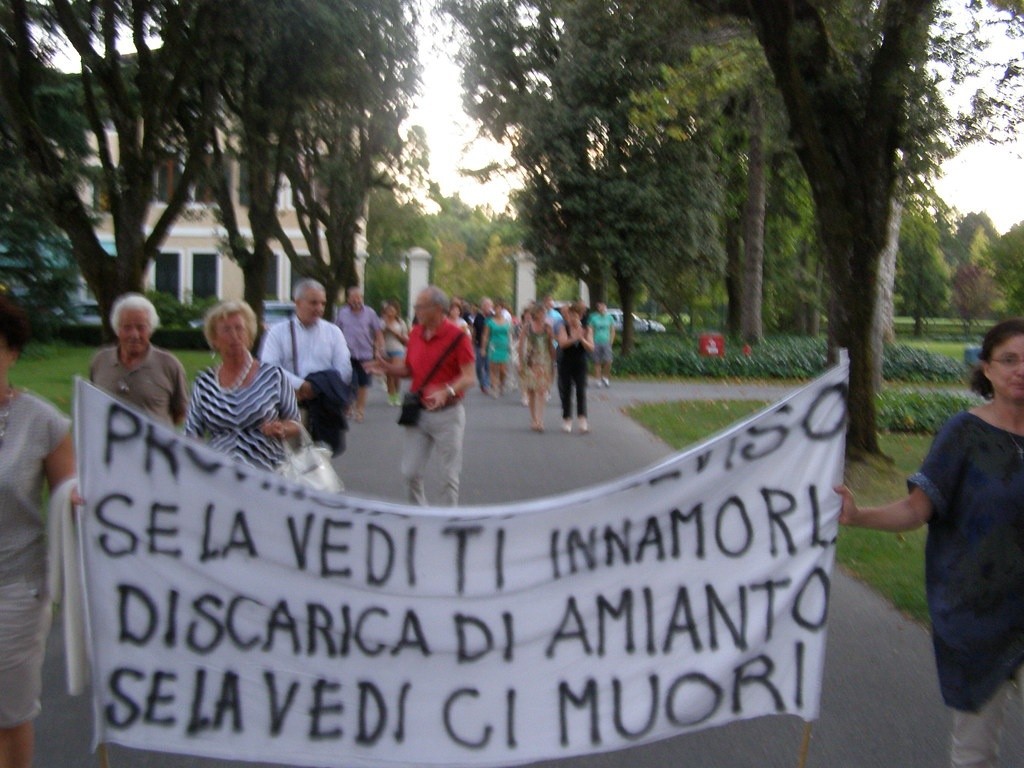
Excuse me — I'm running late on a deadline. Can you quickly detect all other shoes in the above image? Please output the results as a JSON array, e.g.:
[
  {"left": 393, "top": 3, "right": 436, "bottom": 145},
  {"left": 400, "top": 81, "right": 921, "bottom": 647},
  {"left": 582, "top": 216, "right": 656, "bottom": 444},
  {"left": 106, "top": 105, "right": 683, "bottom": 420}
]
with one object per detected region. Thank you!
[
  {"left": 560, "top": 422, "right": 572, "bottom": 433},
  {"left": 345, "top": 408, "right": 354, "bottom": 418},
  {"left": 355, "top": 411, "right": 364, "bottom": 422},
  {"left": 601, "top": 375, "right": 610, "bottom": 387},
  {"left": 388, "top": 393, "right": 402, "bottom": 407},
  {"left": 594, "top": 380, "right": 601, "bottom": 388},
  {"left": 578, "top": 420, "right": 587, "bottom": 432},
  {"left": 530, "top": 421, "right": 545, "bottom": 432}
]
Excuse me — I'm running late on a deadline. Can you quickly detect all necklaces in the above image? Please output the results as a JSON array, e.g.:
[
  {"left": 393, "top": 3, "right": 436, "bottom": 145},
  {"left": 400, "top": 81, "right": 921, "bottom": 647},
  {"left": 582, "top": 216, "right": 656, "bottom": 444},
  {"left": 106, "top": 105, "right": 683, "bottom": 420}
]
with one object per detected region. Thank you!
[
  {"left": 214, "top": 350, "right": 254, "bottom": 394},
  {"left": 0, "top": 386, "right": 15, "bottom": 444}
]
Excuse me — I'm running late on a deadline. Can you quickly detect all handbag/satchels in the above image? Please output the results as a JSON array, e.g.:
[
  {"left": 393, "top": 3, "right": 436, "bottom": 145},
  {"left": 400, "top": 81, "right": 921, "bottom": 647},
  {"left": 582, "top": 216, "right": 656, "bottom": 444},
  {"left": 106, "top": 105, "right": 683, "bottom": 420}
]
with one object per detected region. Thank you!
[
  {"left": 274, "top": 420, "right": 346, "bottom": 494},
  {"left": 396, "top": 392, "right": 420, "bottom": 426}
]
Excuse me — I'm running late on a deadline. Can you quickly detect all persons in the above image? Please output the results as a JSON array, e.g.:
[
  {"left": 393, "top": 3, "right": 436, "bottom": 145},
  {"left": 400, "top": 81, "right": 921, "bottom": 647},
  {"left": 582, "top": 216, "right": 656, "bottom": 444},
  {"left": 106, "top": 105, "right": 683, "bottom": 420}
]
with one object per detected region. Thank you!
[
  {"left": 0, "top": 297, "right": 82, "bottom": 768},
  {"left": 374, "top": 293, "right": 617, "bottom": 431},
  {"left": 184, "top": 299, "right": 301, "bottom": 473},
  {"left": 333, "top": 284, "right": 386, "bottom": 421},
  {"left": 362, "top": 286, "right": 475, "bottom": 506},
  {"left": 88, "top": 293, "right": 187, "bottom": 437},
  {"left": 834, "top": 317, "right": 1024, "bottom": 768},
  {"left": 261, "top": 280, "right": 352, "bottom": 433}
]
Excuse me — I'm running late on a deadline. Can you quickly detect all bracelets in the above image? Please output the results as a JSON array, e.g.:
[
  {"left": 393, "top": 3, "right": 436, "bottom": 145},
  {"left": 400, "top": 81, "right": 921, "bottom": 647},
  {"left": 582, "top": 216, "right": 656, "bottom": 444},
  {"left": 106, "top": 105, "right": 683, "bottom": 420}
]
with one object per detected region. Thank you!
[{"left": 446, "top": 385, "right": 456, "bottom": 398}]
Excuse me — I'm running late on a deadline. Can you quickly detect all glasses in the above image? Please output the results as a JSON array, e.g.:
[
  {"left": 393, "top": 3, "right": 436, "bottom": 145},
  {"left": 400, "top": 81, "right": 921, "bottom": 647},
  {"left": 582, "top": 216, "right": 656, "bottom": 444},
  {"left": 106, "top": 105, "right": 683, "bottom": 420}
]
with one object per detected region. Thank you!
[{"left": 990, "top": 356, "right": 1024, "bottom": 368}]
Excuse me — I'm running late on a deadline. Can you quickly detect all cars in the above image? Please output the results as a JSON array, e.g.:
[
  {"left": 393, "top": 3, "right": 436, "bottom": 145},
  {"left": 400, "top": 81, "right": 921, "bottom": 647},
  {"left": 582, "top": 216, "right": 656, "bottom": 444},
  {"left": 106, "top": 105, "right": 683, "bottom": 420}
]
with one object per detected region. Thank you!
[
  {"left": 44, "top": 295, "right": 297, "bottom": 329},
  {"left": 553, "top": 300, "right": 667, "bottom": 332}
]
